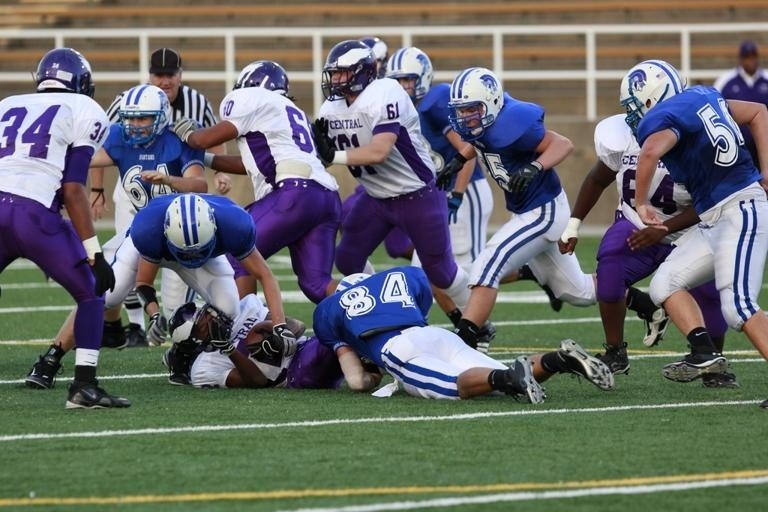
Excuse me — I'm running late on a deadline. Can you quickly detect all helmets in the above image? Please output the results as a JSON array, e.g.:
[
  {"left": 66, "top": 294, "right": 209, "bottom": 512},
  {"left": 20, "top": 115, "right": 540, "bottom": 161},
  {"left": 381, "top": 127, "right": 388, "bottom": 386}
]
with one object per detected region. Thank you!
[
  {"left": 167, "top": 301, "right": 232, "bottom": 351},
  {"left": 447, "top": 66, "right": 504, "bottom": 138},
  {"left": 118, "top": 85, "right": 172, "bottom": 146},
  {"left": 320, "top": 36, "right": 434, "bottom": 100},
  {"left": 35, "top": 48, "right": 94, "bottom": 97},
  {"left": 232, "top": 58, "right": 291, "bottom": 99},
  {"left": 163, "top": 194, "right": 218, "bottom": 268},
  {"left": 618, "top": 60, "right": 681, "bottom": 133}
]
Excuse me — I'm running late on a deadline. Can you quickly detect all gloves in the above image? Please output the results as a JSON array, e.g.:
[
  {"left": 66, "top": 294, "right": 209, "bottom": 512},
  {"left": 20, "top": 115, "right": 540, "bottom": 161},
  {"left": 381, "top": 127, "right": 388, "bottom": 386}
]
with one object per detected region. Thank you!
[
  {"left": 88, "top": 253, "right": 116, "bottom": 296},
  {"left": 146, "top": 313, "right": 169, "bottom": 345},
  {"left": 209, "top": 321, "right": 234, "bottom": 354},
  {"left": 507, "top": 162, "right": 540, "bottom": 201},
  {"left": 312, "top": 116, "right": 337, "bottom": 164},
  {"left": 170, "top": 112, "right": 198, "bottom": 139},
  {"left": 434, "top": 153, "right": 467, "bottom": 192},
  {"left": 256, "top": 322, "right": 299, "bottom": 363},
  {"left": 445, "top": 192, "right": 462, "bottom": 225}
]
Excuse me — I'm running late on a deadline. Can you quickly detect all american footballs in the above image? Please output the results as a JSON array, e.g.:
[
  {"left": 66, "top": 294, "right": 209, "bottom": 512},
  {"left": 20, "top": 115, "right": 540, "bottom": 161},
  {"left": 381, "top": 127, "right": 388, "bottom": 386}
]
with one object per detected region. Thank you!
[{"left": 246, "top": 321, "right": 273, "bottom": 353}]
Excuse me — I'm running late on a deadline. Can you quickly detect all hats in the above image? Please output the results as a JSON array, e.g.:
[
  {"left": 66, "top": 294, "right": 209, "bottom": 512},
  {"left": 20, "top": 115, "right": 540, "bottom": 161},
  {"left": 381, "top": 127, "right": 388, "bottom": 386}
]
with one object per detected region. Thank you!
[
  {"left": 740, "top": 42, "right": 758, "bottom": 61},
  {"left": 149, "top": 48, "right": 182, "bottom": 76}
]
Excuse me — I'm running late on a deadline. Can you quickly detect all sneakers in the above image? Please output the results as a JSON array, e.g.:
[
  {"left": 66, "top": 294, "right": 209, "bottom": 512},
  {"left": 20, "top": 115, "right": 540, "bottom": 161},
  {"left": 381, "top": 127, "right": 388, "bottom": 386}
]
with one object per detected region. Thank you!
[
  {"left": 508, "top": 356, "right": 547, "bottom": 405},
  {"left": 103, "top": 322, "right": 150, "bottom": 349},
  {"left": 25, "top": 354, "right": 60, "bottom": 388},
  {"left": 65, "top": 381, "right": 131, "bottom": 409},
  {"left": 643, "top": 305, "right": 670, "bottom": 348},
  {"left": 661, "top": 351, "right": 739, "bottom": 389},
  {"left": 532, "top": 271, "right": 564, "bottom": 311},
  {"left": 561, "top": 340, "right": 631, "bottom": 391},
  {"left": 475, "top": 321, "right": 497, "bottom": 354}
]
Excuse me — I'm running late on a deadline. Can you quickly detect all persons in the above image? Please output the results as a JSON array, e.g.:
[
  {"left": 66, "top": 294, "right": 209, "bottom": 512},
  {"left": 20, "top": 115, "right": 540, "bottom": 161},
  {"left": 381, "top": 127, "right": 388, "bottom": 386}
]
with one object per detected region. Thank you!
[{"left": 1, "top": 37, "right": 768, "bottom": 408}]
